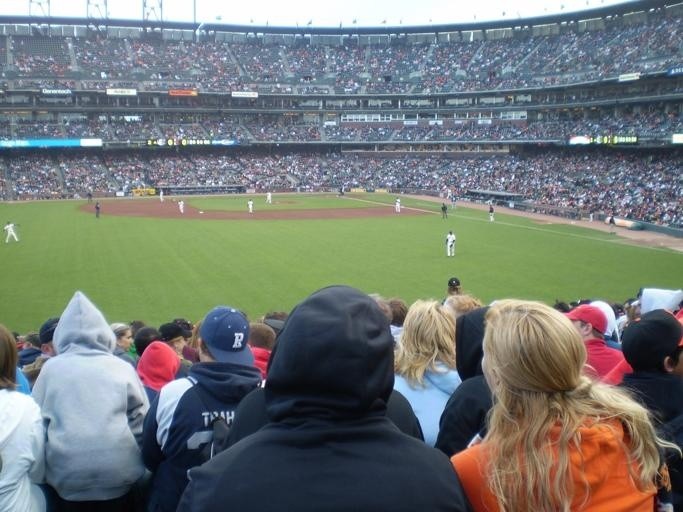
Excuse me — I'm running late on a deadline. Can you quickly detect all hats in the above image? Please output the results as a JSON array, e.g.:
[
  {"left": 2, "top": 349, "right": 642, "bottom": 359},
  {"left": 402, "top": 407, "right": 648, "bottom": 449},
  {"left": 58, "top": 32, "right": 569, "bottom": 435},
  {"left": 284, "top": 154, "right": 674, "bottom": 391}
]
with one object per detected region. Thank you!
[
  {"left": 39, "top": 317, "right": 61, "bottom": 343},
  {"left": 448, "top": 277, "right": 460, "bottom": 286},
  {"left": 197, "top": 306, "right": 255, "bottom": 368},
  {"left": 620, "top": 308, "right": 683, "bottom": 361},
  {"left": 159, "top": 323, "right": 192, "bottom": 343},
  {"left": 561, "top": 304, "right": 608, "bottom": 335}
]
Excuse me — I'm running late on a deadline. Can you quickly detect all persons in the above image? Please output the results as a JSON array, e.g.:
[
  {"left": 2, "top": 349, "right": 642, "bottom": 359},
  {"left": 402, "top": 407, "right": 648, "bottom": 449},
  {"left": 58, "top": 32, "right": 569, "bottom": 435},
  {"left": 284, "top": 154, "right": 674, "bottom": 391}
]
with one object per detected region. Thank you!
[
  {"left": 440, "top": 203, "right": 448, "bottom": 220},
  {"left": 445, "top": 230, "right": 456, "bottom": 256},
  {"left": 84, "top": 190, "right": 93, "bottom": 206},
  {"left": 176, "top": 199, "right": 184, "bottom": 216},
  {"left": 521, "top": 202, "right": 682, "bottom": 235},
  {"left": 246, "top": 199, "right": 253, "bottom": 216},
  {"left": 2, "top": 220, "right": 20, "bottom": 244},
  {"left": 488, "top": 204, "right": 494, "bottom": 222},
  {"left": 94, "top": 202, "right": 101, "bottom": 217},
  {"left": 158, "top": 187, "right": 164, "bottom": 203},
  {"left": 264, "top": 190, "right": 272, "bottom": 205},
  {"left": 394, "top": 197, "right": 400, "bottom": 213},
  {"left": 450, "top": 195, "right": 456, "bottom": 209}
]
